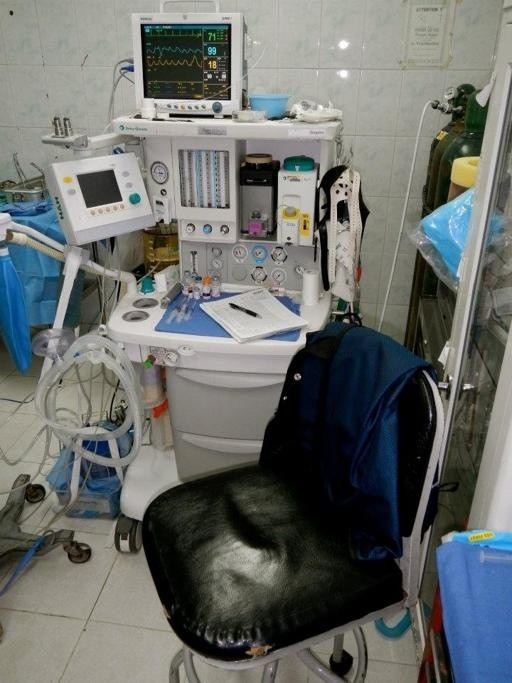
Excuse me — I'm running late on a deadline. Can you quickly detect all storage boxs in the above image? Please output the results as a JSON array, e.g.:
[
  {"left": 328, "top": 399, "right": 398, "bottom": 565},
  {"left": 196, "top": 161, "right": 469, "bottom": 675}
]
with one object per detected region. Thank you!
[{"left": 55, "top": 422, "right": 134, "bottom": 520}]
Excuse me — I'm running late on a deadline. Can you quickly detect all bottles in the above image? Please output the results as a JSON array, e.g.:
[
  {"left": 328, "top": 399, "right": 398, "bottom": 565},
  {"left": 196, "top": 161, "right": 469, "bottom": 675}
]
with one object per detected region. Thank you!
[{"left": 180, "top": 269, "right": 222, "bottom": 300}]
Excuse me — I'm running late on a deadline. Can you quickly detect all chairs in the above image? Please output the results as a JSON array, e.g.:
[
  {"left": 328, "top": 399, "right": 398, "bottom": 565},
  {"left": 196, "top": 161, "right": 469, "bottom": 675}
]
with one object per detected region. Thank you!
[{"left": 141, "top": 322, "right": 445, "bottom": 683}]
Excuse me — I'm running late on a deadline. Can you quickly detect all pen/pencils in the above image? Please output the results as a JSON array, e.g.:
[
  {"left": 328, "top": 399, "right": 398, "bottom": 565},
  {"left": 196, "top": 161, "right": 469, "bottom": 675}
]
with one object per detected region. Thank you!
[
  {"left": 313, "top": 237, "right": 317, "bottom": 263},
  {"left": 228, "top": 301, "right": 263, "bottom": 321}
]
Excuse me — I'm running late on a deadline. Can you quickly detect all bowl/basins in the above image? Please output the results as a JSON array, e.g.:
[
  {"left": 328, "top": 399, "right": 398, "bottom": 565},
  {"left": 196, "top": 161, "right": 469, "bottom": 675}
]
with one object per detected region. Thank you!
[{"left": 247, "top": 93, "right": 290, "bottom": 118}]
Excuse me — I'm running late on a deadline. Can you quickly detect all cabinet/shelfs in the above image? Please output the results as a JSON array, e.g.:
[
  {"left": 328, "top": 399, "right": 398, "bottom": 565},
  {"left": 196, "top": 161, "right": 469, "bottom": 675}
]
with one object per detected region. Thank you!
[
  {"left": 0, "top": 198, "right": 107, "bottom": 338},
  {"left": 417, "top": 540, "right": 512, "bottom": 683}
]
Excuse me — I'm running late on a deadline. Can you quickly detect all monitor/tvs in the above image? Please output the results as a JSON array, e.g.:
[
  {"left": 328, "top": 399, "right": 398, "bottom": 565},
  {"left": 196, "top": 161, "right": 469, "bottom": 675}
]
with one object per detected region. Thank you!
[{"left": 132, "top": 13, "right": 244, "bottom": 120}]
